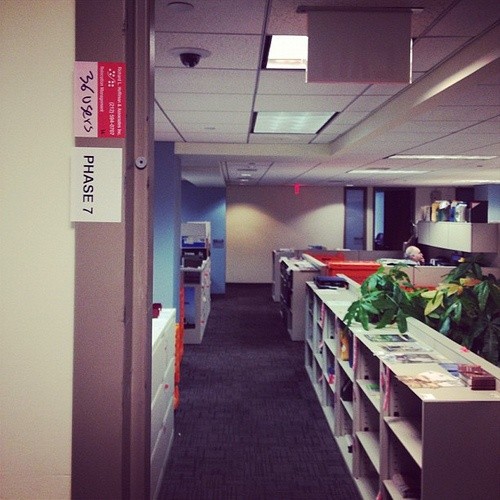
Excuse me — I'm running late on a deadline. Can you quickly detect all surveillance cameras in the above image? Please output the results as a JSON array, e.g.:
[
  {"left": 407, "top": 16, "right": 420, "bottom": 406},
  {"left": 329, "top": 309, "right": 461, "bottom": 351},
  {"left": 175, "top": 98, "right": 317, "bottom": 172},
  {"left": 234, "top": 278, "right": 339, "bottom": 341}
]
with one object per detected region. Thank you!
[{"left": 173, "top": 48, "right": 210, "bottom": 67}]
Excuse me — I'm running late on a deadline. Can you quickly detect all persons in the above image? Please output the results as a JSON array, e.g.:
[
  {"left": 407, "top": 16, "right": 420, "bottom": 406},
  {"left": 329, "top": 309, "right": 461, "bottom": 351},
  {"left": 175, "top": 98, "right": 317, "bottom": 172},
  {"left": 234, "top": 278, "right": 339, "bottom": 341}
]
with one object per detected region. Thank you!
[{"left": 404, "top": 247, "right": 424, "bottom": 266}]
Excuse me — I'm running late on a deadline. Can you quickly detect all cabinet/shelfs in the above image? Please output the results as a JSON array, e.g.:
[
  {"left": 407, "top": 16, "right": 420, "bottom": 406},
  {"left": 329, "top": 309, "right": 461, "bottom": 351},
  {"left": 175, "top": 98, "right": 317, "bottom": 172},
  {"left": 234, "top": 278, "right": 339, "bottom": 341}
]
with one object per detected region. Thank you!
[
  {"left": 303, "top": 274, "right": 500, "bottom": 500},
  {"left": 279, "top": 256, "right": 318, "bottom": 342}
]
